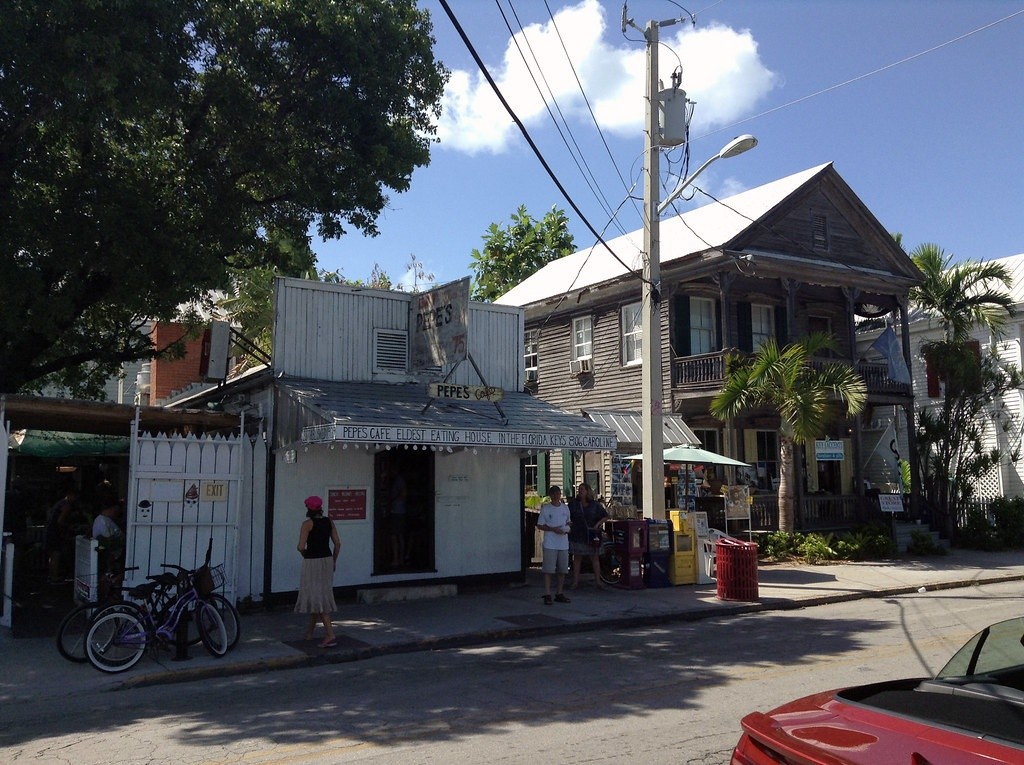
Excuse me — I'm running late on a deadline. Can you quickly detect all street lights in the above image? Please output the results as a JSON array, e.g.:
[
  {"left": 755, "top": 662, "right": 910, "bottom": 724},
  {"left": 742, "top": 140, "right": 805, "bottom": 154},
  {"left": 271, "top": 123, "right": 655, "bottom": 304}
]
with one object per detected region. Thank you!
[{"left": 640, "top": 134, "right": 760, "bottom": 521}]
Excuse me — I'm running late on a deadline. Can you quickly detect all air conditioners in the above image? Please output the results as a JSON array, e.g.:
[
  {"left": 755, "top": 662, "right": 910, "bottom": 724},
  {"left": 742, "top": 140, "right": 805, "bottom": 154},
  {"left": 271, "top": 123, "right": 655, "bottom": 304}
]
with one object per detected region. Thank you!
[{"left": 569, "top": 360, "right": 592, "bottom": 374}]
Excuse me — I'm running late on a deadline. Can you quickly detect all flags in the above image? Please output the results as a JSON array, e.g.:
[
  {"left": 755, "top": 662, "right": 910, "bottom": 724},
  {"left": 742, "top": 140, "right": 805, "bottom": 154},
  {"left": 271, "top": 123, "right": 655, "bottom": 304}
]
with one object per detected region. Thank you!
[
  {"left": 871, "top": 325, "right": 911, "bottom": 385},
  {"left": 875, "top": 420, "right": 903, "bottom": 498}
]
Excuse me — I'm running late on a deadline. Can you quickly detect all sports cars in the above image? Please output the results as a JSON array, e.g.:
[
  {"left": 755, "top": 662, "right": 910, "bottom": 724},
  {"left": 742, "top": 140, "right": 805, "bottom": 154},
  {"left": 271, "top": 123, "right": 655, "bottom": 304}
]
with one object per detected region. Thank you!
[{"left": 729, "top": 617, "right": 1024, "bottom": 765}]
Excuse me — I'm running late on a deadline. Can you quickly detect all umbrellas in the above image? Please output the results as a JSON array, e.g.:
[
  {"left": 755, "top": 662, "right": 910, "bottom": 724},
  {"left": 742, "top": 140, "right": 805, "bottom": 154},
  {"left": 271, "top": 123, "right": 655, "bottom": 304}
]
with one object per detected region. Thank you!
[{"left": 619, "top": 442, "right": 752, "bottom": 510}]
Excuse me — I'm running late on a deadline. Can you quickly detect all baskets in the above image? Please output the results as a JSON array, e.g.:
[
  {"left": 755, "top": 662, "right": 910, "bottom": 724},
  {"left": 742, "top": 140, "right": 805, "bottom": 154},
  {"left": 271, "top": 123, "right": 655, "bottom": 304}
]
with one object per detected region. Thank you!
[{"left": 210, "top": 563, "right": 228, "bottom": 590}]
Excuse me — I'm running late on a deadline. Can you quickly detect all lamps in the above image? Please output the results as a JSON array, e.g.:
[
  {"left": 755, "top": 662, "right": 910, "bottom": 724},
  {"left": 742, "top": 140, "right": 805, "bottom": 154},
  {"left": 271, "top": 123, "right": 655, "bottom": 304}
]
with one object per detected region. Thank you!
[{"left": 207, "top": 394, "right": 235, "bottom": 411}]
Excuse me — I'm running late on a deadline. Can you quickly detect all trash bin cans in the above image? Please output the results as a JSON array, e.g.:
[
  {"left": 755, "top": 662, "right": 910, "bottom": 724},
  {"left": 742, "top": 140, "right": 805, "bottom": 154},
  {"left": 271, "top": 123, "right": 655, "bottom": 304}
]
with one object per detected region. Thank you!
[{"left": 715, "top": 537, "right": 759, "bottom": 602}]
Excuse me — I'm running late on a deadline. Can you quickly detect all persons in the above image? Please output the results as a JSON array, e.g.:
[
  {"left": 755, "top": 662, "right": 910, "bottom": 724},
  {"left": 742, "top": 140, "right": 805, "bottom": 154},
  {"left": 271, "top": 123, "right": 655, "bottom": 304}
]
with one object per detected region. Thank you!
[
  {"left": 2, "top": 474, "right": 126, "bottom": 601},
  {"left": 536, "top": 485, "right": 571, "bottom": 605},
  {"left": 295, "top": 496, "right": 340, "bottom": 648},
  {"left": 567, "top": 483, "right": 610, "bottom": 590},
  {"left": 387, "top": 465, "right": 425, "bottom": 567}
]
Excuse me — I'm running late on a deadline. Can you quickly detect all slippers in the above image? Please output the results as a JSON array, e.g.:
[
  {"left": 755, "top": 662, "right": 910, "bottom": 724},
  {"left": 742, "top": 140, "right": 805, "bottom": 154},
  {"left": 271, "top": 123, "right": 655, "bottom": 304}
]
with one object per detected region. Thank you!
[
  {"left": 317, "top": 640, "right": 337, "bottom": 648},
  {"left": 303, "top": 633, "right": 314, "bottom": 641}
]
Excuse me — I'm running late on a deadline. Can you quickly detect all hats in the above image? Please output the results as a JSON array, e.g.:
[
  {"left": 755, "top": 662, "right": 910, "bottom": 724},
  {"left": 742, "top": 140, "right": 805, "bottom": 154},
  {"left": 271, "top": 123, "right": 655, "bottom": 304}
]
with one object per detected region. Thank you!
[
  {"left": 304, "top": 496, "right": 322, "bottom": 511},
  {"left": 101, "top": 497, "right": 123, "bottom": 508}
]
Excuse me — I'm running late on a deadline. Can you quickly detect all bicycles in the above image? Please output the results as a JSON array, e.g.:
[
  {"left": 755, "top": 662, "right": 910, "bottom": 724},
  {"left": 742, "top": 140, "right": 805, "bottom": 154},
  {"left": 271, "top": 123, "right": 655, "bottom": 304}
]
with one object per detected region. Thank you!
[
  {"left": 595, "top": 527, "right": 626, "bottom": 585},
  {"left": 56, "top": 538, "right": 240, "bottom": 675}
]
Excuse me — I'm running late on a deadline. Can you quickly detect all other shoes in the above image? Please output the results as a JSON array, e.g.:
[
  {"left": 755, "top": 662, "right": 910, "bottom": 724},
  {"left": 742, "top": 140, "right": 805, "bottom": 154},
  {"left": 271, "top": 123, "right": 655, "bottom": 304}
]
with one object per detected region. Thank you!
[
  {"left": 567, "top": 582, "right": 576, "bottom": 589},
  {"left": 403, "top": 555, "right": 410, "bottom": 566},
  {"left": 554, "top": 593, "right": 571, "bottom": 603},
  {"left": 595, "top": 582, "right": 610, "bottom": 592},
  {"left": 542, "top": 594, "right": 552, "bottom": 605},
  {"left": 389, "top": 560, "right": 401, "bottom": 570}
]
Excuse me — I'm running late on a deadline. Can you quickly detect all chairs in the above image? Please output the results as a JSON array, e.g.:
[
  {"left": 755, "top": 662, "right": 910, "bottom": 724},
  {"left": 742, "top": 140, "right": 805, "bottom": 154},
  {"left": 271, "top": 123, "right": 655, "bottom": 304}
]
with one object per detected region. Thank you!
[{"left": 942, "top": 677, "right": 1024, "bottom": 707}]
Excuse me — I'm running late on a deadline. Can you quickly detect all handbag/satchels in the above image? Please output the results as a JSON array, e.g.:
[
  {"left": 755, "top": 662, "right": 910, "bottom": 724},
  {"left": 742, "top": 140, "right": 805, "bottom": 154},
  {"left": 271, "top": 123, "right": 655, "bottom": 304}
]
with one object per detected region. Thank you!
[
  {"left": 103, "top": 572, "right": 123, "bottom": 603},
  {"left": 587, "top": 527, "right": 603, "bottom": 546}
]
